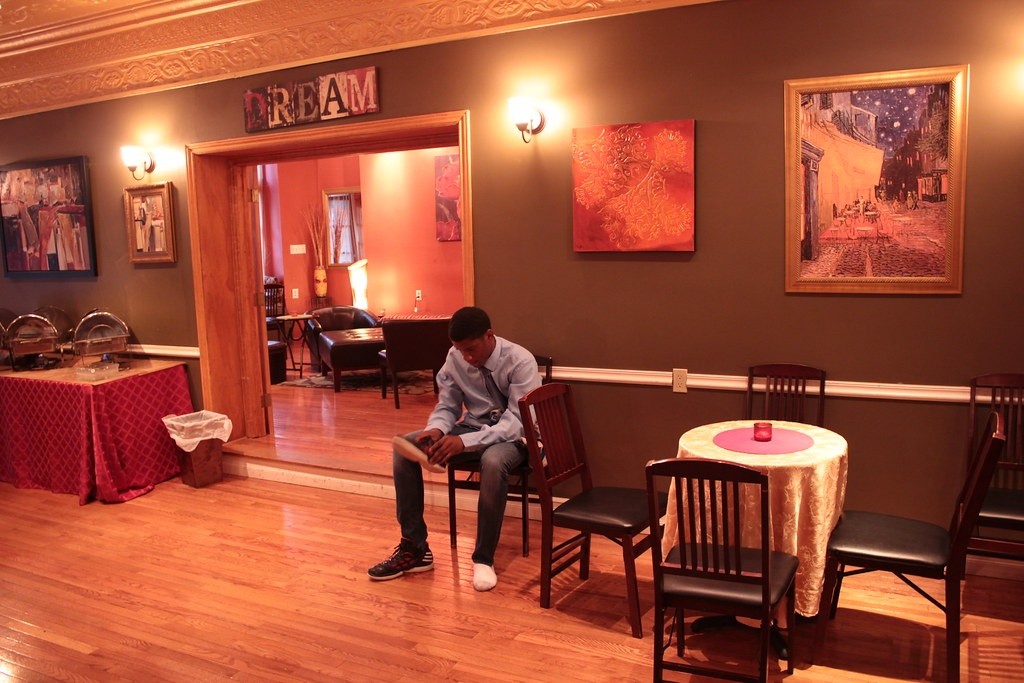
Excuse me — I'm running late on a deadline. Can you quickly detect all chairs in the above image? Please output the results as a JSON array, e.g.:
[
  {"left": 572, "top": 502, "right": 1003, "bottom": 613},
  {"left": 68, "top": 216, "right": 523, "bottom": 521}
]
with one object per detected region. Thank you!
[
  {"left": 645, "top": 457, "right": 799, "bottom": 683},
  {"left": 448, "top": 353, "right": 554, "bottom": 557},
  {"left": 264, "top": 284, "right": 288, "bottom": 359},
  {"left": 813, "top": 374, "right": 1024, "bottom": 683},
  {"left": 747, "top": 363, "right": 827, "bottom": 427},
  {"left": 517, "top": 382, "right": 669, "bottom": 639}
]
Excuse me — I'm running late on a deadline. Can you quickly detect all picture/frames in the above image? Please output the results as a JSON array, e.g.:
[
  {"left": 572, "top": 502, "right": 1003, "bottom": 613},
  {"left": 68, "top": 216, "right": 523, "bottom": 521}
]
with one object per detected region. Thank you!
[
  {"left": 123, "top": 180, "right": 177, "bottom": 264},
  {"left": 0, "top": 154, "right": 97, "bottom": 279},
  {"left": 784, "top": 64, "right": 969, "bottom": 296}
]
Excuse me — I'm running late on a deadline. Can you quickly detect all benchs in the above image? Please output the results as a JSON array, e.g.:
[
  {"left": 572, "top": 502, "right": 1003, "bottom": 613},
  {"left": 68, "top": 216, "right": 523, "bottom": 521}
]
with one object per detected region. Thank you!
[
  {"left": 377, "top": 314, "right": 454, "bottom": 409},
  {"left": 319, "top": 327, "right": 384, "bottom": 392}
]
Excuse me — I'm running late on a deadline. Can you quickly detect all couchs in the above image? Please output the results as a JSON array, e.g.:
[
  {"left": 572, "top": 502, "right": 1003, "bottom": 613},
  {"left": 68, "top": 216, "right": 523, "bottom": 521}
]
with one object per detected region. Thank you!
[{"left": 304, "top": 304, "right": 384, "bottom": 372}]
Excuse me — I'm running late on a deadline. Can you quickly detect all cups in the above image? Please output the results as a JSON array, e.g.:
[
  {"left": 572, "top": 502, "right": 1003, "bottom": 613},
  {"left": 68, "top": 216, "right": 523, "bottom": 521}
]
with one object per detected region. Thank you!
[{"left": 754, "top": 422, "right": 773, "bottom": 442}]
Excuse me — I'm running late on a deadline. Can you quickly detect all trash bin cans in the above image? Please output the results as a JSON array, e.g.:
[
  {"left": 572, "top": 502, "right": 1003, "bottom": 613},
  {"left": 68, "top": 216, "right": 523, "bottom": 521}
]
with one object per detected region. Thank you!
[{"left": 164, "top": 410, "right": 225, "bottom": 487}]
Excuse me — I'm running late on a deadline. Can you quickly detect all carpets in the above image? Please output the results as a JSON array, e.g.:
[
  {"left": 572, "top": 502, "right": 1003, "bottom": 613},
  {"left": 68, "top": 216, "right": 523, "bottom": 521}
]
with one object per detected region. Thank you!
[{"left": 278, "top": 370, "right": 434, "bottom": 395}]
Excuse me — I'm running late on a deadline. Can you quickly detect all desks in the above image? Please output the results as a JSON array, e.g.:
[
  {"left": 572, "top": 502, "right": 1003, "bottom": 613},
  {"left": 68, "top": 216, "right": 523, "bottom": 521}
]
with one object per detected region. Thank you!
[
  {"left": 662, "top": 420, "right": 848, "bottom": 660},
  {"left": 311, "top": 297, "right": 329, "bottom": 310},
  {"left": 0, "top": 350, "right": 194, "bottom": 505},
  {"left": 276, "top": 314, "right": 321, "bottom": 378}
]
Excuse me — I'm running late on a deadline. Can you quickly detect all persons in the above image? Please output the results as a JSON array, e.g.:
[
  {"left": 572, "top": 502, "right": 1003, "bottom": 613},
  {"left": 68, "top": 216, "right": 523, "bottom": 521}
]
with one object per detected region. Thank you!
[{"left": 367, "top": 305, "right": 546, "bottom": 591}]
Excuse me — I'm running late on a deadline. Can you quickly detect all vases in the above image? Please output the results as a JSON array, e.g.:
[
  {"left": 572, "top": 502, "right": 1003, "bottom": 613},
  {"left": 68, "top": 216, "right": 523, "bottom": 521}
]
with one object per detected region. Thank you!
[{"left": 314, "top": 265, "right": 327, "bottom": 297}]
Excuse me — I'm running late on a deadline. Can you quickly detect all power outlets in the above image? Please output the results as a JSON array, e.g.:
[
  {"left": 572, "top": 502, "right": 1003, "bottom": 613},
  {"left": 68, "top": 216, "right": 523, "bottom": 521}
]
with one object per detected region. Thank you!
[
  {"left": 673, "top": 368, "right": 688, "bottom": 394},
  {"left": 416, "top": 290, "right": 422, "bottom": 300}
]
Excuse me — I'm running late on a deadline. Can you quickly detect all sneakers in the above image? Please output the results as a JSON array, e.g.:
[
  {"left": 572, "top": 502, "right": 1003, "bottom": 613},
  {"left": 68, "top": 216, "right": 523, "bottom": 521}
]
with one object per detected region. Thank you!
[
  {"left": 367, "top": 537, "right": 434, "bottom": 580},
  {"left": 391, "top": 434, "right": 446, "bottom": 473}
]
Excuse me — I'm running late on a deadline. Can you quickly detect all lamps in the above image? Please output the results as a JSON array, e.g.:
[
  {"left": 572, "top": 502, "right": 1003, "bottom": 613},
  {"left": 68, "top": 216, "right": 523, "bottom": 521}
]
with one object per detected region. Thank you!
[
  {"left": 121, "top": 145, "right": 155, "bottom": 180},
  {"left": 510, "top": 98, "right": 545, "bottom": 143}
]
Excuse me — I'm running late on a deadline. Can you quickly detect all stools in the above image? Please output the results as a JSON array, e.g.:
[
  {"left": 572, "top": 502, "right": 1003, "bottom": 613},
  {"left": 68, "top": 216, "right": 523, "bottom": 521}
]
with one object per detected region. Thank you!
[{"left": 267, "top": 340, "right": 287, "bottom": 384}]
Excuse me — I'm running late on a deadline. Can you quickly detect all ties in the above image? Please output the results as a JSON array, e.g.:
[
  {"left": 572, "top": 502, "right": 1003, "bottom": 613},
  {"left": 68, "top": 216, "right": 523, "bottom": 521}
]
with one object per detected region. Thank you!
[{"left": 480, "top": 365, "right": 509, "bottom": 414}]
